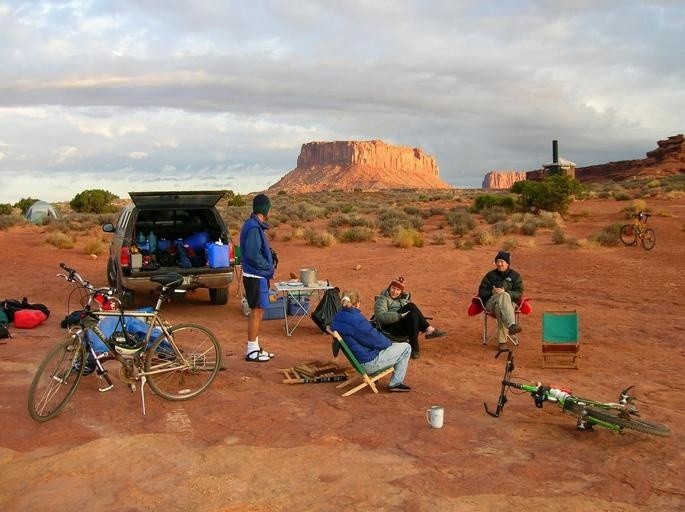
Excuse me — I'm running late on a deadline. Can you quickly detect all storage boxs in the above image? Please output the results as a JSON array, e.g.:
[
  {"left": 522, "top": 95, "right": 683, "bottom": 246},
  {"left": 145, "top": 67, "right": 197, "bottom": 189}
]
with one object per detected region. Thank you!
[
  {"left": 277, "top": 295, "right": 287, "bottom": 302},
  {"left": 286, "top": 294, "right": 304, "bottom": 299},
  {"left": 262, "top": 302, "right": 284, "bottom": 320}
]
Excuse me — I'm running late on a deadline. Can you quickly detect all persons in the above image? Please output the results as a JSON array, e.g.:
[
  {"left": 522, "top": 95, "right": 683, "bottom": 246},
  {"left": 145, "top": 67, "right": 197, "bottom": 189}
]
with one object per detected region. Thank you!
[
  {"left": 373, "top": 277, "right": 448, "bottom": 358},
  {"left": 240, "top": 194, "right": 275, "bottom": 362},
  {"left": 479, "top": 250, "right": 524, "bottom": 351},
  {"left": 331, "top": 286, "right": 413, "bottom": 392}
]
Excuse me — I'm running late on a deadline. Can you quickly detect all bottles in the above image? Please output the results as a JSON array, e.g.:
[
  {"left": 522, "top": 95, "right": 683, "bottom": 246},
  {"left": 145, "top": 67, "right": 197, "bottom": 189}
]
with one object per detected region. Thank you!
[{"left": 549, "top": 388, "right": 572, "bottom": 402}]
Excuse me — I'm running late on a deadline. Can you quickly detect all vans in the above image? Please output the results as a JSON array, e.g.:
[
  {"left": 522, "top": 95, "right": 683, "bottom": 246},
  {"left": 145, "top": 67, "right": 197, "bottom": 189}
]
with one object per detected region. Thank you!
[{"left": 101, "top": 190, "right": 234, "bottom": 305}]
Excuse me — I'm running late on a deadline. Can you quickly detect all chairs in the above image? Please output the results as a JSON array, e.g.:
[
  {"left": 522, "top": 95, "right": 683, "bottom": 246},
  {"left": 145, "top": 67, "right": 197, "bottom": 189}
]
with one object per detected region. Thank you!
[
  {"left": 293, "top": 361, "right": 349, "bottom": 376},
  {"left": 542, "top": 310, "right": 580, "bottom": 370},
  {"left": 370, "top": 314, "right": 409, "bottom": 343},
  {"left": 468, "top": 295, "right": 533, "bottom": 346},
  {"left": 276, "top": 368, "right": 351, "bottom": 384},
  {"left": 326, "top": 325, "right": 394, "bottom": 397}
]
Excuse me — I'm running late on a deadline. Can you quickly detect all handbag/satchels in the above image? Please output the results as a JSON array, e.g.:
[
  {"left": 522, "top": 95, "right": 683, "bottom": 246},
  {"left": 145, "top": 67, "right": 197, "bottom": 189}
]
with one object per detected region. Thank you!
[
  {"left": 1, "top": 296, "right": 51, "bottom": 323},
  {"left": 62, "top": 310, "right": 98, "bottom": 328},
  {"left": 0, "top": 309, "right": 13, "bottom": 338}
]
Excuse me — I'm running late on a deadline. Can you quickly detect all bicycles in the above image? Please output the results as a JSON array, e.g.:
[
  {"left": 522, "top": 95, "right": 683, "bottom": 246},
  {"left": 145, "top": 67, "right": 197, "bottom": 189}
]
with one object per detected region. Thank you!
[
  {"left": 484, "top": 346, "right": 675, "bottom": 440},
  {"left": 25, "top": 262, "right": 223, "bottom": 423},
  {"left": 620, "top": 209, "right": 657, "bottom": 251}
]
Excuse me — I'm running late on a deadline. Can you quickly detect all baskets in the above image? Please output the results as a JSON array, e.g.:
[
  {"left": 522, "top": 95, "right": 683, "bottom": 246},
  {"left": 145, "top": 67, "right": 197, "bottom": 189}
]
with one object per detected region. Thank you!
[{"left": 263, "top": 297, "right": 285, "bottom": 320}]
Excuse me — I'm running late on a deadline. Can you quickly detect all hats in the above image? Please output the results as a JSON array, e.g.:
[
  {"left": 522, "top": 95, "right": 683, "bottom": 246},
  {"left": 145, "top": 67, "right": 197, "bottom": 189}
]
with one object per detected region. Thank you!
[
  {"left": 252, "top": 194, "right": 271, "bottom": 217},
  {"left": 495, "top": 251, "right": 511, "bottom": 265},
  {"left": 388, "top": 274, "right": 406, "bottom": 293}
]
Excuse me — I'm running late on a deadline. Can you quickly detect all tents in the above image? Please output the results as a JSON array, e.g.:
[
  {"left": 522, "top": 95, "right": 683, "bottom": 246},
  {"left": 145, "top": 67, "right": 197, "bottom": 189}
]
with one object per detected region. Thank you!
[{"left": 24, "top": 200, "right": 61, "bottom": 224}]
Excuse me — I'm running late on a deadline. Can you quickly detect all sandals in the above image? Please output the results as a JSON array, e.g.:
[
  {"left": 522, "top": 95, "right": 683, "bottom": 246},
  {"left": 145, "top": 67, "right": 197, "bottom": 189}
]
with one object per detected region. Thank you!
[
  {"left": 245, "top": 351, "right": 270, "bottom": 361},
  {"left": 260, "top": 348, "right": 275, "bottom": 358}
]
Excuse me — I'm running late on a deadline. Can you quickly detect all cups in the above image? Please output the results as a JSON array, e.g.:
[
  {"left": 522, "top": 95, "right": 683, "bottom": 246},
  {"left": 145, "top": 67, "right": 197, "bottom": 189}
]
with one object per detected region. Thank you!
[{"left": 426, "top": 405, "right": 444, "bottom": 429}]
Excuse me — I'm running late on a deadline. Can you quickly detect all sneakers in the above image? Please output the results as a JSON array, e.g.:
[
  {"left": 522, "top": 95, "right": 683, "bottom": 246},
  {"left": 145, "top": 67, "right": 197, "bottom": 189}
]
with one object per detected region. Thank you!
[
  {"left": 499, "top": 343, "right": 508, "bottom": 351},
  {"left": 508, "top": 323, "right": 522, "bottom": 335},
  {"left": 388, "top": 383, "right": 411, "bottom": 391},
  {"left": 411, "top": 351, "right": 420, "bottom": 359},
  {"left": 425, "top": 328, "right": 447, "bottom": 339}
]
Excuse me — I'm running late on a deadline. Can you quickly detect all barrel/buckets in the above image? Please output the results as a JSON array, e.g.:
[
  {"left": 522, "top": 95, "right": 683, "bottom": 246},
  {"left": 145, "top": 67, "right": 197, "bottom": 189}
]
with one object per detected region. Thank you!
[
  {"left": 300, "top": 267, "right": 316, "bottom": 288},
  {"left": 157, "top": 238, "right": 171, "bottom": 251},
  {"left": 173, "top": 238, "right": 185, "bottom": 256},
  {"left": 207, "top": 239, "right": 230, "bottom": 268},
  {"left": 136, "top": 239, "right": 150, "bottom": 253}
]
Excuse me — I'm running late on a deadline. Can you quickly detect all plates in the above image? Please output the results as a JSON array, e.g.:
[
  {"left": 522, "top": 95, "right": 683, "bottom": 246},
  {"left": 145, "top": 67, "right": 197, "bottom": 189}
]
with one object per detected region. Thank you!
[{"left": 288, "top": 283, "right": 303, "bottom": 286}]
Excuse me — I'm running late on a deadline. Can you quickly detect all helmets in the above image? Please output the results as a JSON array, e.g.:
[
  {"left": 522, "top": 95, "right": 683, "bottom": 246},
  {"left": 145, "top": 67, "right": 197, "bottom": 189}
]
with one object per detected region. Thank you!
[{"left": 110, "top": 330, "right": 143, "bottom": 355}]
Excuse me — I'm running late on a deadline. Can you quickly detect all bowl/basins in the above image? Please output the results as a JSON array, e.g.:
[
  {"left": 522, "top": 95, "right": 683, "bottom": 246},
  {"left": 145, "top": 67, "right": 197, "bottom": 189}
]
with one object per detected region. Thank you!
[{"left": 279, "top": 282, "right": 287, "bottom": 285}]
